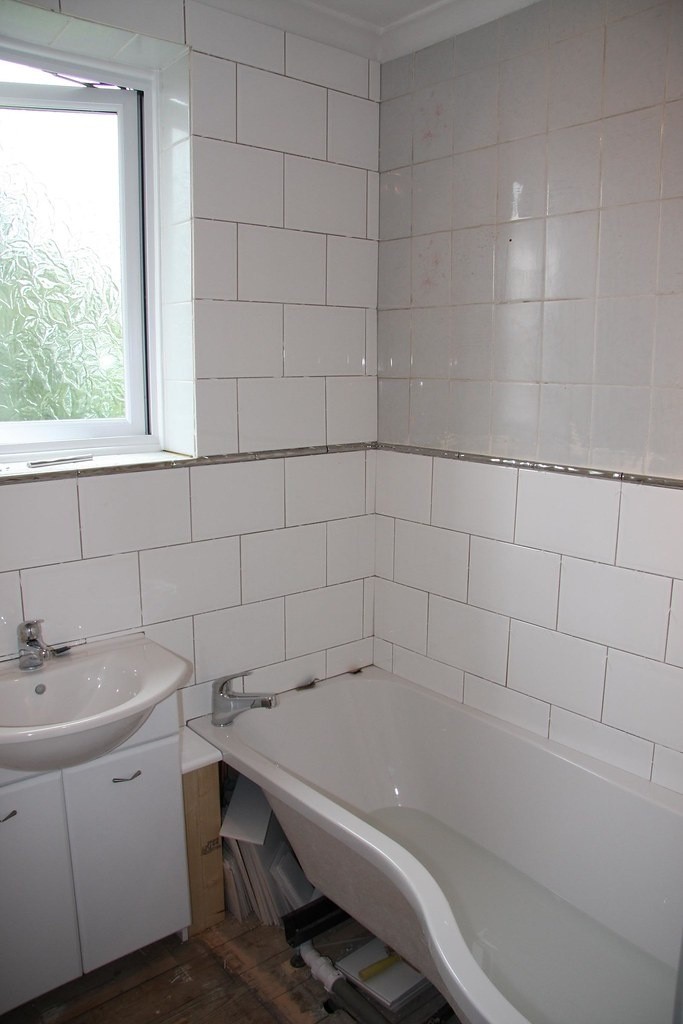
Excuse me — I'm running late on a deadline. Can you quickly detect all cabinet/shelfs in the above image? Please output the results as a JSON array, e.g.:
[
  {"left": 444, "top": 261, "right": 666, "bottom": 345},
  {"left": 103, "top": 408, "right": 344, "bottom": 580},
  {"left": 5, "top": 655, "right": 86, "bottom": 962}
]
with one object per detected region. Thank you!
[{"left": 0, "top": 691, "right": 192, "bottom": 1016}]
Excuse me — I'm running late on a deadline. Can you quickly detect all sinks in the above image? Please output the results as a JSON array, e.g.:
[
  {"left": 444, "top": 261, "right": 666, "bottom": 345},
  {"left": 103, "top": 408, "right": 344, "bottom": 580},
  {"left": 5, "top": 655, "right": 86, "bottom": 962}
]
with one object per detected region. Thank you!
[{"left": 2, "top": 634, "right": 188, "bottom": 772}]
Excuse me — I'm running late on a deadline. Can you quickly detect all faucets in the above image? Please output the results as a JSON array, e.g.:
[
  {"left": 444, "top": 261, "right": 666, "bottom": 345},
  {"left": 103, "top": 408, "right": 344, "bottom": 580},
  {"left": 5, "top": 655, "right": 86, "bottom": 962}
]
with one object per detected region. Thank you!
[
  {"left": 18, "top": 619, "right": 53, "bottom": 669},
  {"left": 212, "top": 670, "right": 277, "bottom": 727}
]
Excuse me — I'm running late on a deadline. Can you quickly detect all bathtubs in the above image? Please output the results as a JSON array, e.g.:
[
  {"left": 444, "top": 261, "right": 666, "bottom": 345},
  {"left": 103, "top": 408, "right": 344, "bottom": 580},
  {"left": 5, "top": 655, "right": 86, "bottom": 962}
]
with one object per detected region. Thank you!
[{"left": 190, "top": 661, "right": 683, "bottom": 1024}]
